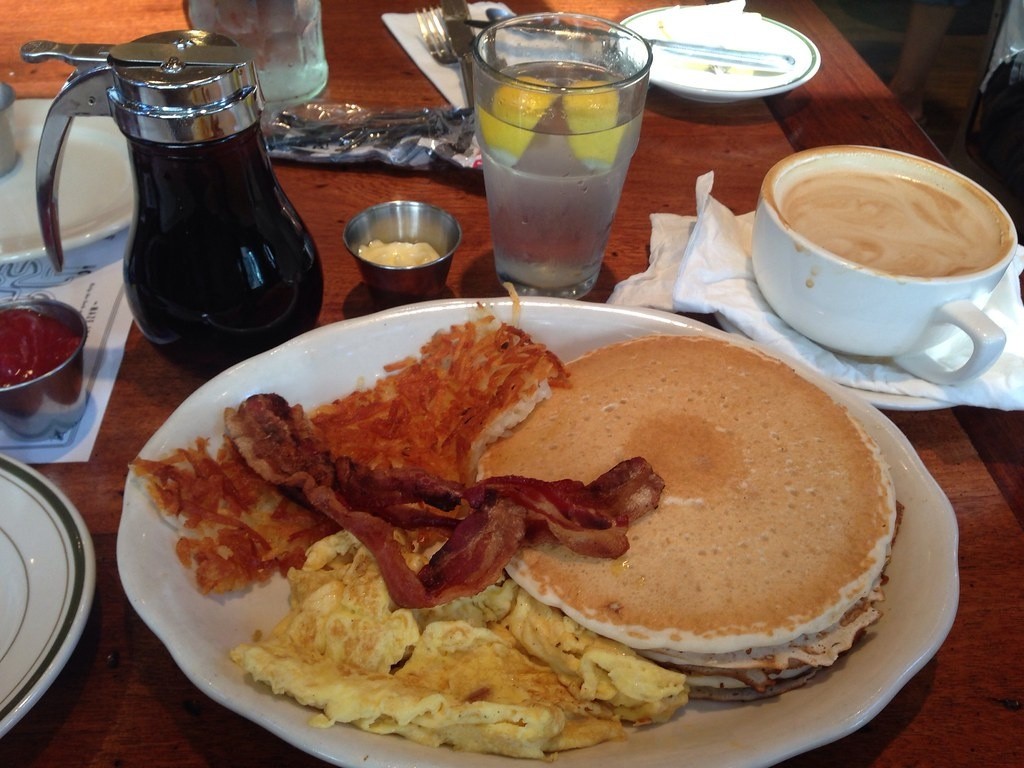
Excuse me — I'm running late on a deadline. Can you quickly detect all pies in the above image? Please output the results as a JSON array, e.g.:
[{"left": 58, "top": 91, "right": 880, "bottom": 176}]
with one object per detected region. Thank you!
[{"left": 475, "top": 334, "right": 907, "bottom": 699}]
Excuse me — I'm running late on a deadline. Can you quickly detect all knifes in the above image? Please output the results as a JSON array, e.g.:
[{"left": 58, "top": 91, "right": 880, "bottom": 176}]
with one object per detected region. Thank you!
[{"left": 440, "top": 2, "right": 473, "bottom": 80}]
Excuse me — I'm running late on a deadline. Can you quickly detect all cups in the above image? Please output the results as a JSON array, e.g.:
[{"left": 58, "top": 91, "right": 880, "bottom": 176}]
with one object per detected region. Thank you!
[
  {"left": 0, "top": 302, "right": 86, "bottom": 438},
  {"left": 343, "top": 201, "right": 462, "bottom": 306},
  {"left": 753, "top": 141, "right": 1018, "bottom": 391},
  {"left": 471, "top": 10, "right": 650, "bottom": 298},
  {"left": 187, "top": 1, "right": 325, "bottom": 109}
]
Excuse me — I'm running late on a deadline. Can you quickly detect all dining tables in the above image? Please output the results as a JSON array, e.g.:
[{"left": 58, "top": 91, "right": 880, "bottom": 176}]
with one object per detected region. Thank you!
[{"left": 0, "top": 1, "right": 1020, "bottom": 766}]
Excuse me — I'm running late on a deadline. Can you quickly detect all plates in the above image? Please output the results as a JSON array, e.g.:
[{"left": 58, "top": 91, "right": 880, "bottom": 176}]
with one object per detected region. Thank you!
[
  {"left": 0, "top": 452, "right": 96, "bottom": 739},
  {"left": 612, "top": 6, "right": 819, "bottom": 105},
  {"left": 3, "top": 94, "right": 141, "bottom": 262},
  {"left": 118, "top": 300, "right": 959, "bottom": 765}
]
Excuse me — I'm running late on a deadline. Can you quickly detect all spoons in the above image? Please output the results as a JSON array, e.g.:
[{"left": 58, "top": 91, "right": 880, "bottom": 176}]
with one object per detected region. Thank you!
[{"left": 486, "top": 6, "right": 513, "bottom": 24}]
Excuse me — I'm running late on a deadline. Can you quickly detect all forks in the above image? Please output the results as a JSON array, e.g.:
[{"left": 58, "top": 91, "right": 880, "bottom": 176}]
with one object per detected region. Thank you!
[{"left": 415, "top": 6, "right": 470, "bottom": 112}]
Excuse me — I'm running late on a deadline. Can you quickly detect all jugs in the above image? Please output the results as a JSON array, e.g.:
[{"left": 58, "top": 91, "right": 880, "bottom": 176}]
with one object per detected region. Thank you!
[{"left": 13, "top": 26, "right": 324, "bottom": 370}]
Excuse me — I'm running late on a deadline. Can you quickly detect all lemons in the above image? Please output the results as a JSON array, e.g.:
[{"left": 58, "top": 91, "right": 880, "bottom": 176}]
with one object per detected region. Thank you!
[{"left": 478, "top": 75, "right": 629, "bottom": 173}]
